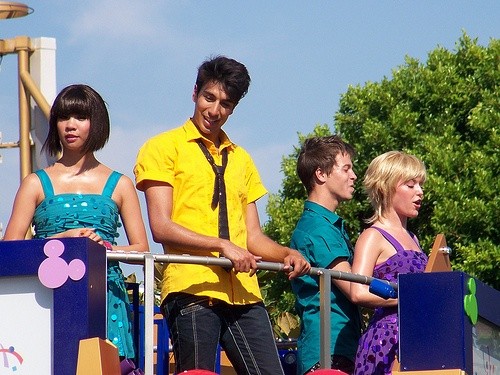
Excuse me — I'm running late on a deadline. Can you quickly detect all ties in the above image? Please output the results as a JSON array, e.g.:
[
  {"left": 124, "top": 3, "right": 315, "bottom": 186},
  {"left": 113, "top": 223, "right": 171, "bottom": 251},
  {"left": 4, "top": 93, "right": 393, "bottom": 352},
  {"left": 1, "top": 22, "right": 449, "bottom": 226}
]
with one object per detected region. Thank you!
[{"left": 194, "top": 138, "right": 234, "bottom": 274}]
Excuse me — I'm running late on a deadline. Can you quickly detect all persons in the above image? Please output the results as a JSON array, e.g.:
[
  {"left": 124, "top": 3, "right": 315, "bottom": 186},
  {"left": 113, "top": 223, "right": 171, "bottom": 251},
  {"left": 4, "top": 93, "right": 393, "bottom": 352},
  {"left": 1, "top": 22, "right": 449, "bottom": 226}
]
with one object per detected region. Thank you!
[
  {"left": 350, "top": 151, "right": 429, "bottom": 375},
  {"left": 2, "top": 86, "right": 150, "bottom": 358},
  {"left": 288, "top": 135, "right": 358, "bottom": 375},
  {"left": 133, "top": 54, "right": 311, "bottom": 375}
]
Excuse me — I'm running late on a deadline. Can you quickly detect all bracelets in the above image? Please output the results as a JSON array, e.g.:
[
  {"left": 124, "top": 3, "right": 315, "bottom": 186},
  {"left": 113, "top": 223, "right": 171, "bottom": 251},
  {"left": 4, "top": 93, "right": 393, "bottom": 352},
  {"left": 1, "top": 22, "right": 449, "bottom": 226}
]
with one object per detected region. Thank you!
[{"left": 103, "top": 241, "right": 112, "bottom": 251}]
